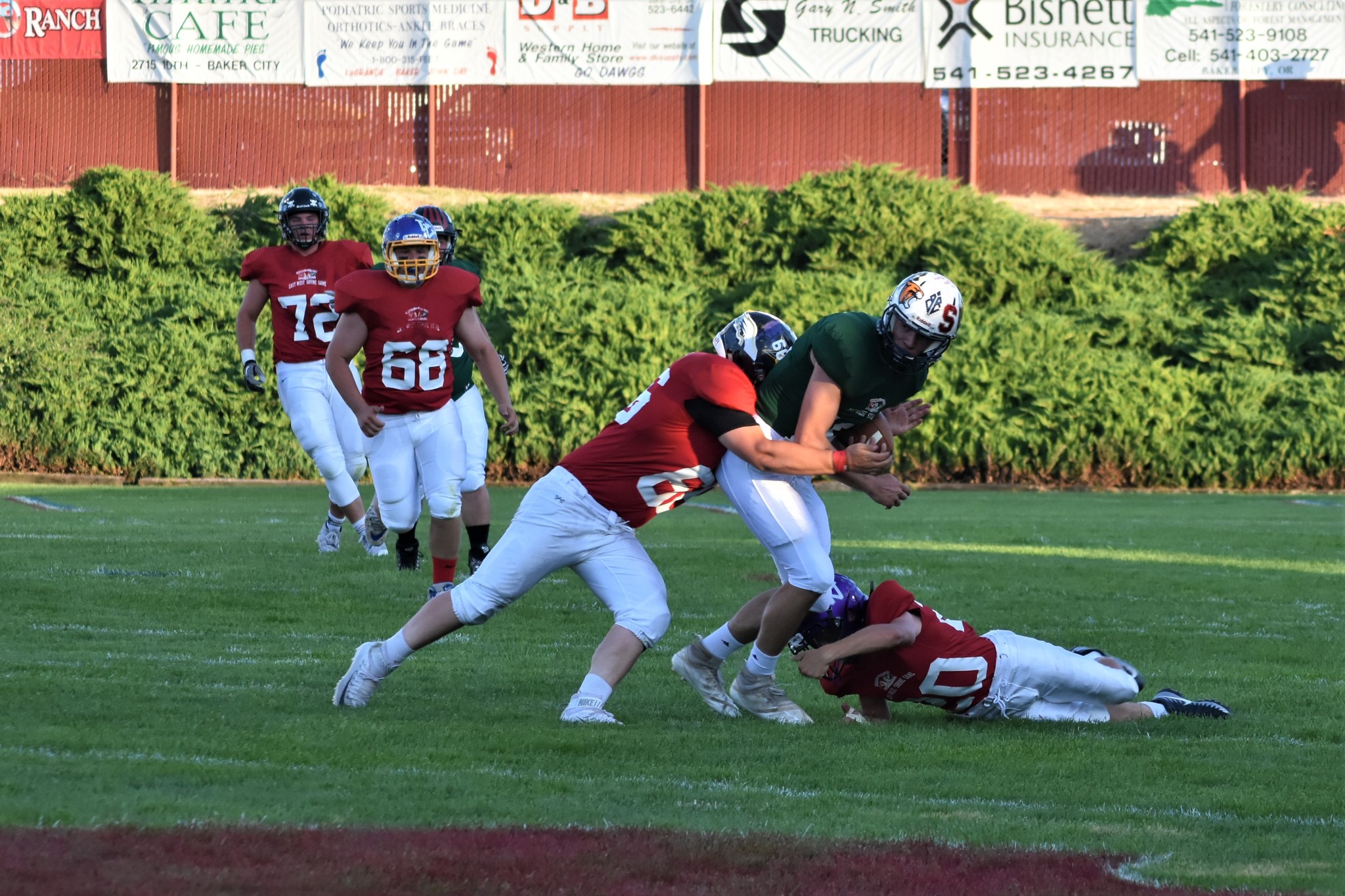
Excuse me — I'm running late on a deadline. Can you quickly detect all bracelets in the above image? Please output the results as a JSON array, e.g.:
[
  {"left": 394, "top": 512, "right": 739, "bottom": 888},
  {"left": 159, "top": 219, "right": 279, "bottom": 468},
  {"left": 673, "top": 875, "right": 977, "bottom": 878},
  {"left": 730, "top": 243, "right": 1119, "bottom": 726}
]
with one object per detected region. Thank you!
[{"left": 832, "top": 450, "right": 849, "bottom": 474}]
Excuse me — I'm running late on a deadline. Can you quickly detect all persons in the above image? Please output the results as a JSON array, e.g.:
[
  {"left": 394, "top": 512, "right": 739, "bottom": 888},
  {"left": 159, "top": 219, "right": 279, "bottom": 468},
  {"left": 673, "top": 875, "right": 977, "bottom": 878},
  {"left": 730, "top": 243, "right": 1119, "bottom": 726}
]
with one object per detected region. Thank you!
[
  {"left": 786, "top": 574, "right": 1233, "bottom": 727},
  {"left": 333, "top": 270, "right": 964, "bottom": 727},
  {"left": 235, "top": 188, "right": 519, "bottom": 603}
]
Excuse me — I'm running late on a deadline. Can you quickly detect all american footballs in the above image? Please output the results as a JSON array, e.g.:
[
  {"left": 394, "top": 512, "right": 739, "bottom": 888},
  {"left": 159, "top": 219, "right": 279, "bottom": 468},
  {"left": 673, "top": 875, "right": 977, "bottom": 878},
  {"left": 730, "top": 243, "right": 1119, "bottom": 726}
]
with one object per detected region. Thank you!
[{"left": 832, "top": 412, "right": 894, "bottom": 457}]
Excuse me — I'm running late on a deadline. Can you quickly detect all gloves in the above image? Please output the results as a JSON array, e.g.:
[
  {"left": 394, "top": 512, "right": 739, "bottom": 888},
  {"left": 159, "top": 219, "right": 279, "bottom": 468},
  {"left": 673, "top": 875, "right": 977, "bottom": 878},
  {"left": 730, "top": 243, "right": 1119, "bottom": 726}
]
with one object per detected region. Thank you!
[
  {"left": 499, "top": 354, "right": 509, "bottom": 376},
  {"left": 243, "top": 360, "right": 266, "bottom": 393}
]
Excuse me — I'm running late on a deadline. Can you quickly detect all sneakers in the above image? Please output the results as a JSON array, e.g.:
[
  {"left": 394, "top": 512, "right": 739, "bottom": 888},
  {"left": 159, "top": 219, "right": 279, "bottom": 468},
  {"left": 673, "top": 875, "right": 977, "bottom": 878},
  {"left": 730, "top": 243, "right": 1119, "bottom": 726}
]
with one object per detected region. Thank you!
[
  {"left": 332, "top": 642, "right": 402, "bottom": 708},
  {"left": 396, "top": 538, "right": 424, "bottom": 572},
  {"left": 1152, "top": 688, "right": 1233, "bottom": 720},
  {"left": 671, "top": 634, "right": 742, "bottom": 718},
  {"left": 1069, "top": 646, "right": 1145, "bottom": 694},
  {"left": 427, "top": 582, "right": 455, "bottom": 600},
  {"left": 560, "top": 693, "right": 624, "bottom": 725},
  {"left": 364, "top": 491, "right": 388, "bottom": 546},
  {"left": 729, "top": 659, "right": 814, "bottom": 726},
  {"left": 358, "top": 532, "right": 388, "bottom": 557},
  {"left": 468, "top": 544, "right": 491, "bottom": 575},
  {"left": 315, "top": 520, "right": 342, "bottom": 555}
]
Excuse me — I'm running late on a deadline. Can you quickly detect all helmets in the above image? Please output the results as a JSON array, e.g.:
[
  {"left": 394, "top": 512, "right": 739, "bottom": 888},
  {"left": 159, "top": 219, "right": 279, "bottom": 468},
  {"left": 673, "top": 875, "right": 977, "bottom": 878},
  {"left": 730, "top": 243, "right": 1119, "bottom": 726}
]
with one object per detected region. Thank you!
[
  {"left": 878, "top": 271, "right": 964, "bottom": 376},
  {"left": 787, "top": 573, "right": 869, "bottom": 682},
  {"left": 273, "top": 188, "right": 330, "bottom": 250},
  {"left": 382, "top": 214, "right": 440, "bottom": 281},
  {"left": 411, "top": 206, "right": 458, "bottom": 265},
  {"left": 712, "top": 310, "right": 798, "bottom": 391}
]
